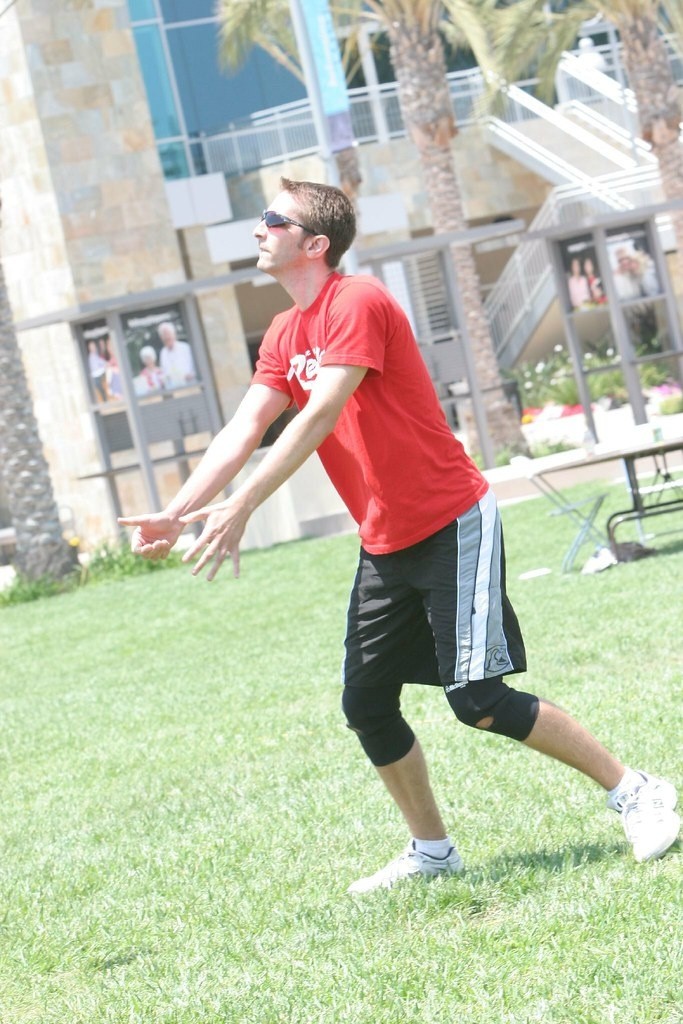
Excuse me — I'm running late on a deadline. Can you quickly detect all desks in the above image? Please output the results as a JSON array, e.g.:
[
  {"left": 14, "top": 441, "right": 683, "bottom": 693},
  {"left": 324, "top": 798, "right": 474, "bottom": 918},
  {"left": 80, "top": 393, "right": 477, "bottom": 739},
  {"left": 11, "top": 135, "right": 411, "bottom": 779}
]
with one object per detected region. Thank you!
[{"left": 536, "top": 435, "right": 683, "bottom": 564}]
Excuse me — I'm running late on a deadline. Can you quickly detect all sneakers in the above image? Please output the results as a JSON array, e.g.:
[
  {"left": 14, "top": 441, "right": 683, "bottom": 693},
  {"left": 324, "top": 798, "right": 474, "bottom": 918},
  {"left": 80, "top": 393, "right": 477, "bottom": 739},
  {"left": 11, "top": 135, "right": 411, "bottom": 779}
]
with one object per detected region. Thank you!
[
  {"left": 348, "top": 839, "right": 462, "bottom": 897},
  {"left": 606, "top": 769, "right": 681, "bottom": 863}
]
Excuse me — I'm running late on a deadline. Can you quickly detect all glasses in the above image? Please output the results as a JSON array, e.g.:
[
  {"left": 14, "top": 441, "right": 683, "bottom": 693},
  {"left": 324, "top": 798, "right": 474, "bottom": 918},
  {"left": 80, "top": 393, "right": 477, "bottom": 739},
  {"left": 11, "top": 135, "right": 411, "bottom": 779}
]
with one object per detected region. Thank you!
[{"left": 261, "top": 209, "right": 318, "bottom": 237}]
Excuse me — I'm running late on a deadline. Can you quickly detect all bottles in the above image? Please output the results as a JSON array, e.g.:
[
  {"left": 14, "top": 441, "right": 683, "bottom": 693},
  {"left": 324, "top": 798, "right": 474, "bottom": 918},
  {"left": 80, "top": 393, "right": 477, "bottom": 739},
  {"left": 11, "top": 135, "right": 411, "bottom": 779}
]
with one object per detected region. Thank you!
[
  {"left": 584, "top": 430, "right": 596, "bottom": 459},
  {"left": 651, "top": 412, "right": 664, "bottom": 445}
]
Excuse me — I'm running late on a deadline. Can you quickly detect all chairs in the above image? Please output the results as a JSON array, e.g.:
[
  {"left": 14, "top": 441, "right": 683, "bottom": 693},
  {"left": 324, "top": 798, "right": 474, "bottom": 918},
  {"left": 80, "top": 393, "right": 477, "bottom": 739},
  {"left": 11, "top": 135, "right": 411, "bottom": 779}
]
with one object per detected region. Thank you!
[{"left": 509, "top": 454, "right": 633, "bottom": 574}]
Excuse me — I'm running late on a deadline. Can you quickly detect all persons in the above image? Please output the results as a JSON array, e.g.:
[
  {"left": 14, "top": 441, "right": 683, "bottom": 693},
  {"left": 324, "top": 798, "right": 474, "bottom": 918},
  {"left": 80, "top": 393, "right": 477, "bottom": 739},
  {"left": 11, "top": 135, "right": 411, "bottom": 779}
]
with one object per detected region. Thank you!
[
  {"left": 567, "top": 256, "right": 606, "bottom": 310},
  {"left": 87, "top": 336, "right": 124, "bottom": 404},
  {"left": 613, "top": 242, "right": 660, "bottom": 300},
  {"left": 117, "top": 177, "right": 680, "bottom": 896},
  {"left": 132, "top": 322, "right": 198, "bottom": 397}
]
